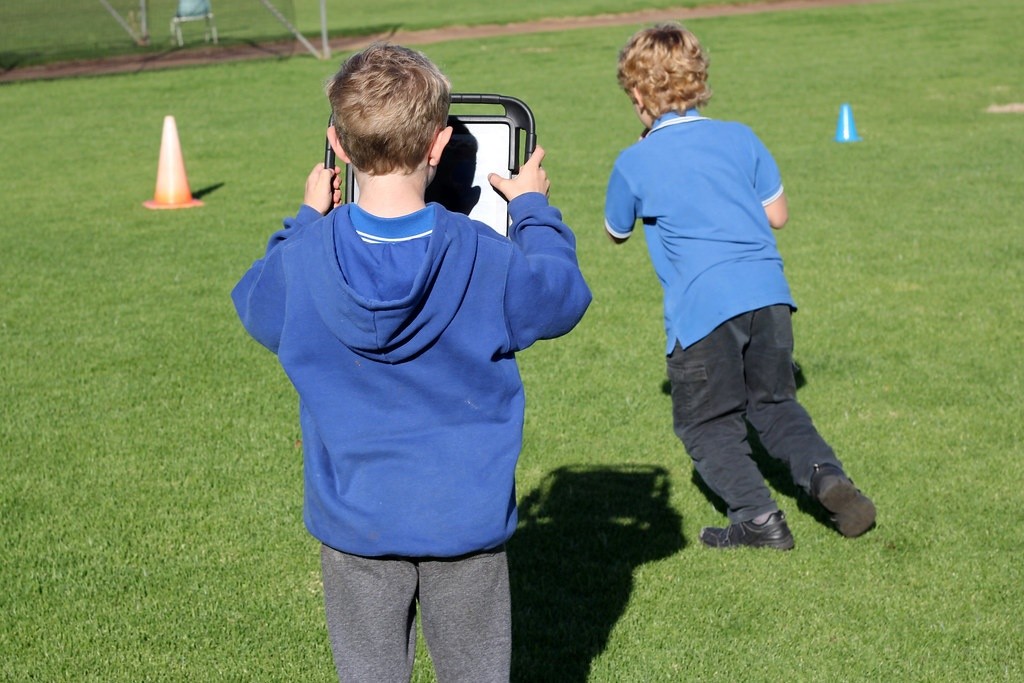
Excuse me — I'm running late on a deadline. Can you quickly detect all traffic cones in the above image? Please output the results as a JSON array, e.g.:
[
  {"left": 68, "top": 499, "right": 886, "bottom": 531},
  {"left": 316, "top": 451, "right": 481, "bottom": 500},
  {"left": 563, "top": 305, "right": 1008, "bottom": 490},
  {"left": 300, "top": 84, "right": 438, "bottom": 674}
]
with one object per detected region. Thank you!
[
  {"left": 140, "top": 113, "right": 206, "bottom": 211},
  {"left": 832, "top": 103, "right": 864, "bottom": 144}
]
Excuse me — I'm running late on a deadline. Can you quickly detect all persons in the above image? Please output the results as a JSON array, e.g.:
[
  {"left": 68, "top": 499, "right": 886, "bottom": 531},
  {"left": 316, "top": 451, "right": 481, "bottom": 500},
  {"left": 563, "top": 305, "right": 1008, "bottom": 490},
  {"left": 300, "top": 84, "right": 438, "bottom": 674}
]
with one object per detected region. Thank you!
[
  {"left": 602, "top": 21, "right": 877, "bottom": 552},
  {"left": 230, "top": 42, "right": 592, "bottom": 682}
]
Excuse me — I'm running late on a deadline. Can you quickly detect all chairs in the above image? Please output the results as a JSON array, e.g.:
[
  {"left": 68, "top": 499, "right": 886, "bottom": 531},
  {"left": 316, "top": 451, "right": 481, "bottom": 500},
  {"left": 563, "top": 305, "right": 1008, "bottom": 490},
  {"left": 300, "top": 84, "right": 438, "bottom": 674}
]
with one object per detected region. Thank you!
[{"left": 170, "top": 0, "right": 218, "bottom": 49}]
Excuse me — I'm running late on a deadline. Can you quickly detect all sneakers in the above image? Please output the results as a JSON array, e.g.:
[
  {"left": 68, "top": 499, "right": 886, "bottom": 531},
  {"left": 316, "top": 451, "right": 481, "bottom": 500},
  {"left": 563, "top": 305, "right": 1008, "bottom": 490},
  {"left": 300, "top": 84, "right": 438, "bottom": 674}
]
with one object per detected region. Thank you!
[
  {"left": 702, "top": 510, "right": 794, "bottom": 551},
  {"left": 810, "top": 461, "right": 876, "bottom": 538}
]
